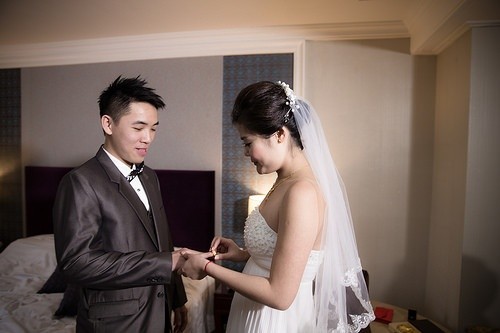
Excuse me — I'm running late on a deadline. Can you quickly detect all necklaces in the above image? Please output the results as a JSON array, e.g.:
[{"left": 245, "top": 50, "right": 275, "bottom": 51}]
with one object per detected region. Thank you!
[{"left": 260, "top": 165, "right": 310, "bottom": 211}]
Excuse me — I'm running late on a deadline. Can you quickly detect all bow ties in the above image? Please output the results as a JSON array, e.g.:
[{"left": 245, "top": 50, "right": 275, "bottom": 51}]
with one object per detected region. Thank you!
[{"left": 126, "top": 161, "right": 144, "bottom": 183}]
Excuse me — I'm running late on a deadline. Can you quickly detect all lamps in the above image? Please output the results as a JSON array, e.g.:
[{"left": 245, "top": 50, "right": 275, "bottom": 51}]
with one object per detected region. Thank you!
[{"left": 248, "top": 195, "right": 266, "bottom": 216}]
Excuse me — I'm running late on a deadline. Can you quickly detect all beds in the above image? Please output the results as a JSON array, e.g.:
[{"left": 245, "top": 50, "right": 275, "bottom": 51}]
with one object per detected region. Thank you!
[{"left": 0, "top": 166, "right": 216, "bottom": 333}]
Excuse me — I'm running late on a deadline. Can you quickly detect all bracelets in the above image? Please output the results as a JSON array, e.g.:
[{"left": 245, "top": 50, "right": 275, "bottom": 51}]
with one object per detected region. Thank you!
[{"left": 204, "top": 260, "right": 214, "bottom": 275}]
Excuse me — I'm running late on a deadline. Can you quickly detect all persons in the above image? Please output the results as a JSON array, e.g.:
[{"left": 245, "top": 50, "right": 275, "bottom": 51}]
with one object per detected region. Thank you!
[
  {"left": 52, "top": 73, "right": 217, "bottom": 333},
  {"left": 179, "top": 80, "right": 331, "bottom": 333}
]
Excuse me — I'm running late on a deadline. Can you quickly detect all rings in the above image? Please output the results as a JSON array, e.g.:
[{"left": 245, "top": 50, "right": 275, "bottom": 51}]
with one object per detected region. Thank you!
[{"left": 211, "top": 250, "right": 217, "bottom": 256}]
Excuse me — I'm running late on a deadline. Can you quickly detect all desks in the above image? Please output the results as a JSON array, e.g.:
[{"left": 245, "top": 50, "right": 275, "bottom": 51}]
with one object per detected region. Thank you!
[{"left": 369, "top": 301, "right": 453, "bottom": 333}]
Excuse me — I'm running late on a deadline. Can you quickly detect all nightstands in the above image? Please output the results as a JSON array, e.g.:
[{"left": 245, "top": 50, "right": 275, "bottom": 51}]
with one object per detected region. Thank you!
[{"left": 215, "top": 281, "right": 235, "bottom": 333}]
